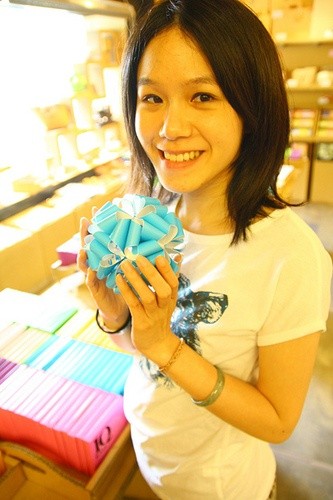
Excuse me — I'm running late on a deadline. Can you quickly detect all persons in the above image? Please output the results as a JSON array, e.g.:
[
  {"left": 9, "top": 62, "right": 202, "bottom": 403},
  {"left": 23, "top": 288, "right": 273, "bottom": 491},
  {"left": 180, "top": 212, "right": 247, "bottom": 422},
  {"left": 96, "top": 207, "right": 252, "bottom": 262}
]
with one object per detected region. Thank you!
[{"left": 76, "top": 0, "right": 333, "bottom": 500}]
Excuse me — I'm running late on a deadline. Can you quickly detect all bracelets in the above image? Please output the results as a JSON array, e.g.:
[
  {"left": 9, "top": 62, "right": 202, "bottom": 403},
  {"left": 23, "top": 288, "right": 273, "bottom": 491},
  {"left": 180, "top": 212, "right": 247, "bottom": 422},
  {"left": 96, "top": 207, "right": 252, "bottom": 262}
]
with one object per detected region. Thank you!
[
  {"left": 158, "top": 338, "right": 185, "bottom": 372},
  {"left": 95, "top": 308, "right": 131, "bottom": 335},
  {"left": 191, "top": 365, "right": 225, "bottom": 407}
]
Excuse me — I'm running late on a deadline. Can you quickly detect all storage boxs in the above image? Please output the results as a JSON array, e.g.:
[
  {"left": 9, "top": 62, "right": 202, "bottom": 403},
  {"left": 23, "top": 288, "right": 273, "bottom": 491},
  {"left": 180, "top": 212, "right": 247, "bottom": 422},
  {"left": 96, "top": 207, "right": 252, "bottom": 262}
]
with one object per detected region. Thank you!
[{"left": 1, "top": 425, "right": 131, "bottom": 500}]
